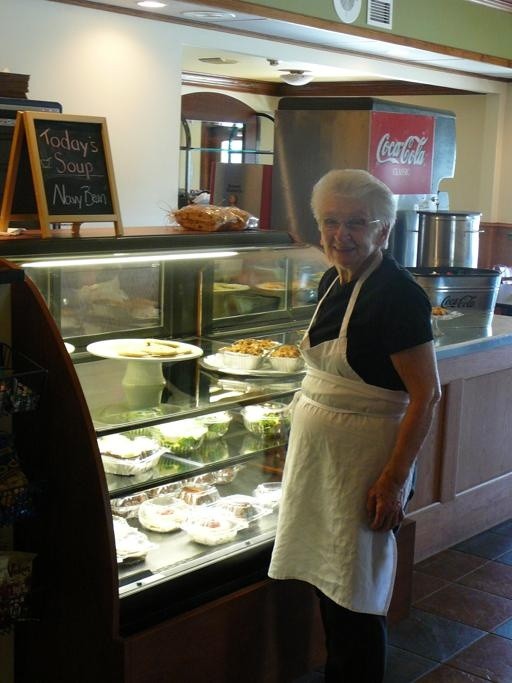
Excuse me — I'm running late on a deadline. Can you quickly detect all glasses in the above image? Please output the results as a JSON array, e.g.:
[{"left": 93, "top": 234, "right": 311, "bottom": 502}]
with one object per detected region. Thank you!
[{"left": 319, "top": 215, "right": 381, "bottom": 233}]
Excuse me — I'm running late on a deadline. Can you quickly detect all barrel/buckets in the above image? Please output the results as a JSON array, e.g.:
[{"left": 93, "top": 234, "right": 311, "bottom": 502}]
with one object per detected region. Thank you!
[
  {"left": 417, "top": 209, "right": 482, "bottom": 268},
  {"left": 405, "top": 266, "right": 502, "bottom": 328}
]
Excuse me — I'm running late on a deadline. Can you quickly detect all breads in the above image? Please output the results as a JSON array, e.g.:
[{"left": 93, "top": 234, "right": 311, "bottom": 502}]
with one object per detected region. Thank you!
[
  {"left": 118, "top": 337, "right": 192, "bottom": 357},
  {"left": 172, "top": 204, "right": 259, "bottom": 233}
]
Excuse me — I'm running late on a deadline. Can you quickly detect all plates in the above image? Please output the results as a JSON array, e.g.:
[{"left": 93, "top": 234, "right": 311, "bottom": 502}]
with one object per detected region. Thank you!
[
  {"left": 213, "top": 282, "right": 249, "bottom": 297},
  {"left": 255, "top": 283, "right": 286, "bottom": 292},
  {"left": 87, "top": 336, "right": 206, "bottom": 366}
]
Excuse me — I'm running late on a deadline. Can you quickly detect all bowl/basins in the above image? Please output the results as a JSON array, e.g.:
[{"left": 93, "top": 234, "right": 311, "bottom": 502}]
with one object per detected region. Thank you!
[{"left": 159, "top": 401, "right": 290, "bottom": 453}]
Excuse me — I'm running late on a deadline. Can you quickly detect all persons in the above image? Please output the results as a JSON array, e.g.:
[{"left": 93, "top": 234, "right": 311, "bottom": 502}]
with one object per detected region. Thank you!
[{"left": 265, "top": 166, "right": 441, "bottom": 678}]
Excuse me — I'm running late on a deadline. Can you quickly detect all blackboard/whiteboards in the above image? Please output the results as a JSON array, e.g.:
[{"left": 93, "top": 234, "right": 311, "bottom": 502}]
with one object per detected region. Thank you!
[
  {"left": 5, "top": 110, "right": 39, "bottom": 223},
  {"left": 24, "top": 111, "right": 122, "bottom": 222}
]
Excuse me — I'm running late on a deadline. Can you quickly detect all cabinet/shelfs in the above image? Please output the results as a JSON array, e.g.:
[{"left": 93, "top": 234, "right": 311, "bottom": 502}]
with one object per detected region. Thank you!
[{"left": 0, "top": 231, "right": 328, "bottom": 683}]
